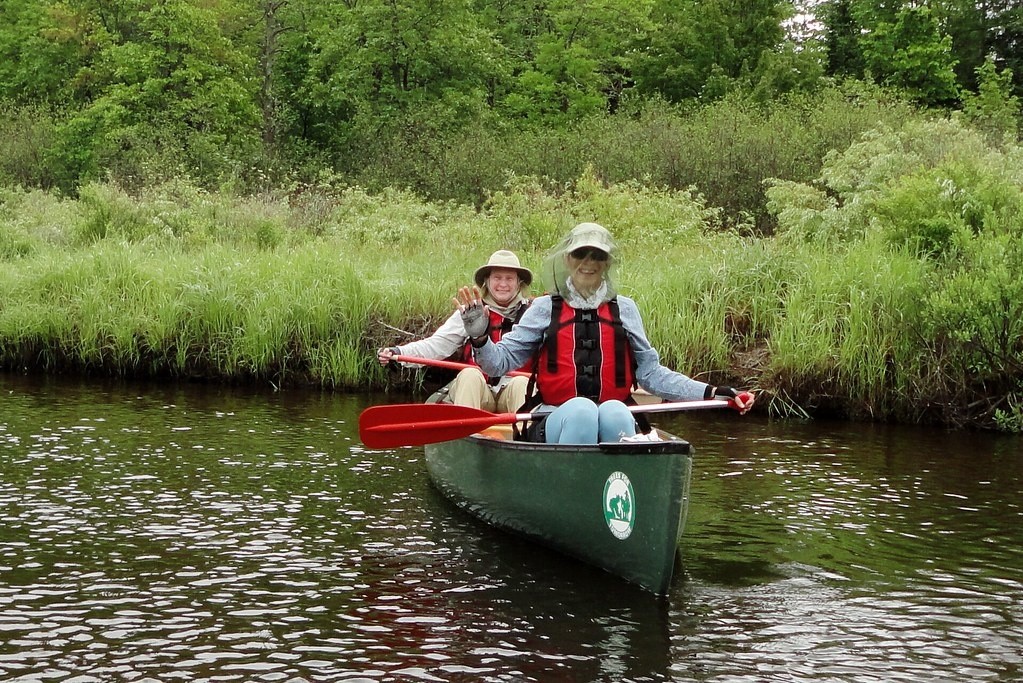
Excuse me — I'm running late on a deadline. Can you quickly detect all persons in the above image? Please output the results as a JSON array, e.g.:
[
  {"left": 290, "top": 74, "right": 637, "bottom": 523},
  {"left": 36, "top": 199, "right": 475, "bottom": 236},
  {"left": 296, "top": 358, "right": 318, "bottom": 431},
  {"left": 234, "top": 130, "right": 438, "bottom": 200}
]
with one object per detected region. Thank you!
[
  {"left": 379, "top": 250, "right": 538, "bottom": 412},
  {"left": 454, "top": 222, "right": 756, "bottom": 443}
]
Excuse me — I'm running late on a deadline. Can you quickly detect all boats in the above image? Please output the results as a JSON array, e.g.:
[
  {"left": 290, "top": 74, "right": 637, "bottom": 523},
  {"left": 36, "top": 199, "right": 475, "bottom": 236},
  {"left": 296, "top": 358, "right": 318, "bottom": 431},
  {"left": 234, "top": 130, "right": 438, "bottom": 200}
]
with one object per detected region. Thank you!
[{"left": 425, "top": 372, "right": 695, "bottom": 601}]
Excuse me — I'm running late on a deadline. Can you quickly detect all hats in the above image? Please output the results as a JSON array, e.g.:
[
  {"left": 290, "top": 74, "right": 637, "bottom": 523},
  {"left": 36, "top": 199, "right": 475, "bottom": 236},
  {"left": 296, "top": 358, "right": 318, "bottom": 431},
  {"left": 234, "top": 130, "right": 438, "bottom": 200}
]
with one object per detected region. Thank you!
[
  {"left": 475, "top": 249, "right": 533, "bottom": 289},
  {"left": 566, "top": 223, "right": 611, "bottom": 255}
]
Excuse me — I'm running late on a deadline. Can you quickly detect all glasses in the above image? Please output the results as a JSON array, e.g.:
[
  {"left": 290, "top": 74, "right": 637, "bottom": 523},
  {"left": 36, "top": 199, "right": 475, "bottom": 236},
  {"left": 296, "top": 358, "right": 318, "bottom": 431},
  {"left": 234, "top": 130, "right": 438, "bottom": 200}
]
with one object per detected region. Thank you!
[{"left": 570, "top": 247, "right": 607, "bottom": 261}]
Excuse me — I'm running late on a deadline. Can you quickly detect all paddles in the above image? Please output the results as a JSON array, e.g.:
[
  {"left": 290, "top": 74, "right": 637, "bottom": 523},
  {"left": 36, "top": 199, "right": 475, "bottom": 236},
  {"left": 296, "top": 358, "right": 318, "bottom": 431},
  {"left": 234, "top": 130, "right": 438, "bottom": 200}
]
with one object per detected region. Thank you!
[
  {"left": 391, "top": 352, "right": 534, "bottom": 379},
  {"left": 357, "top": 393, "right": 758, "bottom": 451}
]
